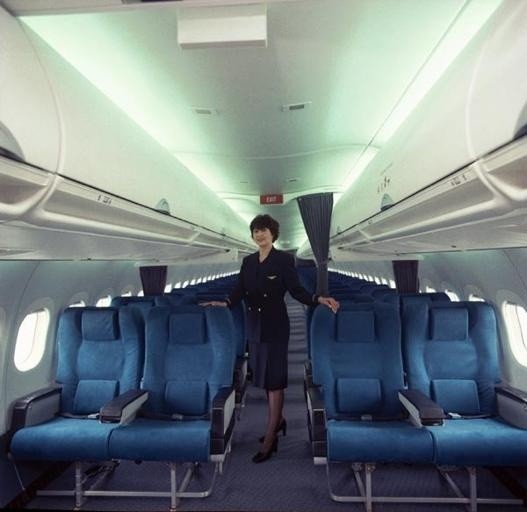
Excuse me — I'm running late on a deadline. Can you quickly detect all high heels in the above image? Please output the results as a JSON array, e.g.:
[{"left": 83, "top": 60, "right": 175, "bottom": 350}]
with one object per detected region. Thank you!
[
  {"left": 252, "top": 436, "right": 278, "bottom": 463},
  {"left": 259, "top": 418, "right": 287, "bottom": 443}
]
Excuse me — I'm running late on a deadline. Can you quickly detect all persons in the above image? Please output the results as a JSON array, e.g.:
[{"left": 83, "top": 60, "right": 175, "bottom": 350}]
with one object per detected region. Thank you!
[{"left": 198, "top": 214, "right": 340, "bottom": 463}]
